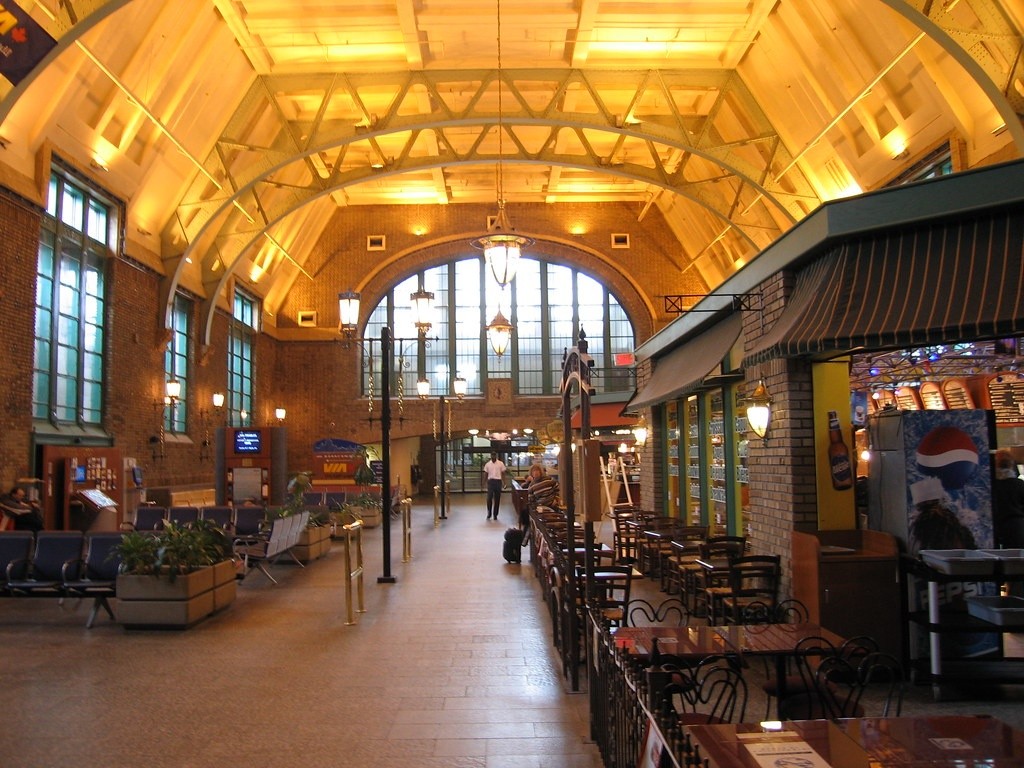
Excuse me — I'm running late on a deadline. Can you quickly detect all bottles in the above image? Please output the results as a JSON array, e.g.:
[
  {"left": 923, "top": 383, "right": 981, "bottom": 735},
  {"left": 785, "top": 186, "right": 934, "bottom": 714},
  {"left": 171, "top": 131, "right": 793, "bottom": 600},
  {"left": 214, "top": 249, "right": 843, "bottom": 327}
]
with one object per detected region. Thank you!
[
  {"left": 827, "top": 411, "right": 852, "bottom": 491},
  {"left": 908, "top": 478, "right": 1001, "bottom": 667}
]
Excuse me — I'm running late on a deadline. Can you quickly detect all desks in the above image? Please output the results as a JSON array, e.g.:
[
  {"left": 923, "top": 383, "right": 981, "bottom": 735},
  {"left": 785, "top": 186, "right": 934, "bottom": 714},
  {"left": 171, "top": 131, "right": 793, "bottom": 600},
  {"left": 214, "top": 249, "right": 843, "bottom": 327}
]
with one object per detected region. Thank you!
[{"left": 539, "top": 513, "right": 1024, "bottom": 768}]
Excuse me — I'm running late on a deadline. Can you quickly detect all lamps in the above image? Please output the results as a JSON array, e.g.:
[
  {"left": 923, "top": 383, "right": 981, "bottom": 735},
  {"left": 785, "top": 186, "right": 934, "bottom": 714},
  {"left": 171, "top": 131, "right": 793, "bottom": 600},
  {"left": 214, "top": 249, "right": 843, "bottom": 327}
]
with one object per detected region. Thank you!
[
  {"left": 153, "top": 379, "right": 180, "bottom": 407},
  {"left": 212, "top": 392, "right": 224, "bottom": 413},
  {"left": 739, "top": 377, "right": 773, "bottom": 447},
  {"left": 470, "top": 1, "right": 534, "bottom": 291},
  {"left": 485, "top": 303, "right": 514, "bottom": 358},
  {"left": 269, "top": 404, "right": 286, "bottom": 425},
  {"left": 630, "top": 416, "right": 647, "bottom": 447}
]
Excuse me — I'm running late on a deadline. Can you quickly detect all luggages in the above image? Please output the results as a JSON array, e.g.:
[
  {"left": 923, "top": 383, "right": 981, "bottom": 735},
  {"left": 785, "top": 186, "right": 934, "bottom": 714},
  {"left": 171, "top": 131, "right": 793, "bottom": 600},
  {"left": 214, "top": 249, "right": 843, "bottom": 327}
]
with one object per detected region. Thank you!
[{"left": 502, "top": 527, "right": 523, "bottom": 564}]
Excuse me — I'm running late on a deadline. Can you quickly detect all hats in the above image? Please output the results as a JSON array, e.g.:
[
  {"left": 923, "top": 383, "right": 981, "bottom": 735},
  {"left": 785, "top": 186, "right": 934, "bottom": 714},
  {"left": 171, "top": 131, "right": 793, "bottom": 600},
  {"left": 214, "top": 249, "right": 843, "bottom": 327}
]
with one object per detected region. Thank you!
[{"left": 491, "top": 451, "right": 496, "bottom": 454}]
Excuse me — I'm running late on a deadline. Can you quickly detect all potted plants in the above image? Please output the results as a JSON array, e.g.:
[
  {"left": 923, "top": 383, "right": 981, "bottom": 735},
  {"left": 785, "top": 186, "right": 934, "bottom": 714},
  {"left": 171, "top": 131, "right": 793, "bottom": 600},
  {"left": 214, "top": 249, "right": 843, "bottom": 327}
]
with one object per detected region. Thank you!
[
  {"left": 271, "top": 479, "right": 381, "bottom": 564},
  {"left": 115, "top": 520, "right": 236, "bottom": 631}
]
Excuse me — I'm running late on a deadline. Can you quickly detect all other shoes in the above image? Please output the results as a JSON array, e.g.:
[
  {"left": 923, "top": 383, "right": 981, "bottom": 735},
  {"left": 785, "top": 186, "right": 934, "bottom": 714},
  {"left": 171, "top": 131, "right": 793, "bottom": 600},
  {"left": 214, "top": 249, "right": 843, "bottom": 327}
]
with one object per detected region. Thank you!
[
  {"left": 521, "top": 539, "right": 528, "bottom": 546},
  {"left": 487, "top": 513, "right": 491, "bottom": 518},
  {"left": 493, "top": 515, "right": 497, "bottom": 520}
]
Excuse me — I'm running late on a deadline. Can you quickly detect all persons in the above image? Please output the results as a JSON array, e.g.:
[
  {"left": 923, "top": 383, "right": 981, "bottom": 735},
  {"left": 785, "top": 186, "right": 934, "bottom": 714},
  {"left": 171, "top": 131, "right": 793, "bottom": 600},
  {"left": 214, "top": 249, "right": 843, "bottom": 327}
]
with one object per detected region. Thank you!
[
  {"left": 481, "top": 451, "right": 507, "bottom": 520},
  {"left": 994, "top": 449, "right": 1024, "bottom": 596},
  {"left": 2, "top": 487, "right": 45, "bottom": 550},
  {"left": 521, "top": 476, "right": 533, "bottom": 489},
  {"left": 528, "top": 464, "right": 559, "bottom": 512}
]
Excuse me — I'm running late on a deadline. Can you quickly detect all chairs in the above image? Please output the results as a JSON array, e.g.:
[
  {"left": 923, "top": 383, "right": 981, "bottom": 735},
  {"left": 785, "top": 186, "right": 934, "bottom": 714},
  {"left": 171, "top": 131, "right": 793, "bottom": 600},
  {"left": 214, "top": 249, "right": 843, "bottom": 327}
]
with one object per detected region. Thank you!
[
  {"left": 523, "top": 502, "right": 902, "bottom": 724},
  {"left": 0, "top": 491, "right": 355, "bottom": 629}
]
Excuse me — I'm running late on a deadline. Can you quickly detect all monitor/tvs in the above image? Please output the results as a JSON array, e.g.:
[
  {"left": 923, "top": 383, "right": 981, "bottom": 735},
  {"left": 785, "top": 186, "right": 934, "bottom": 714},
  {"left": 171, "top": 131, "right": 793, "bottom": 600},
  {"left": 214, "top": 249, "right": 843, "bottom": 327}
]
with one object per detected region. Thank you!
[{"left": 234, "top": 430, "right": 261, "bottom": 454}]
